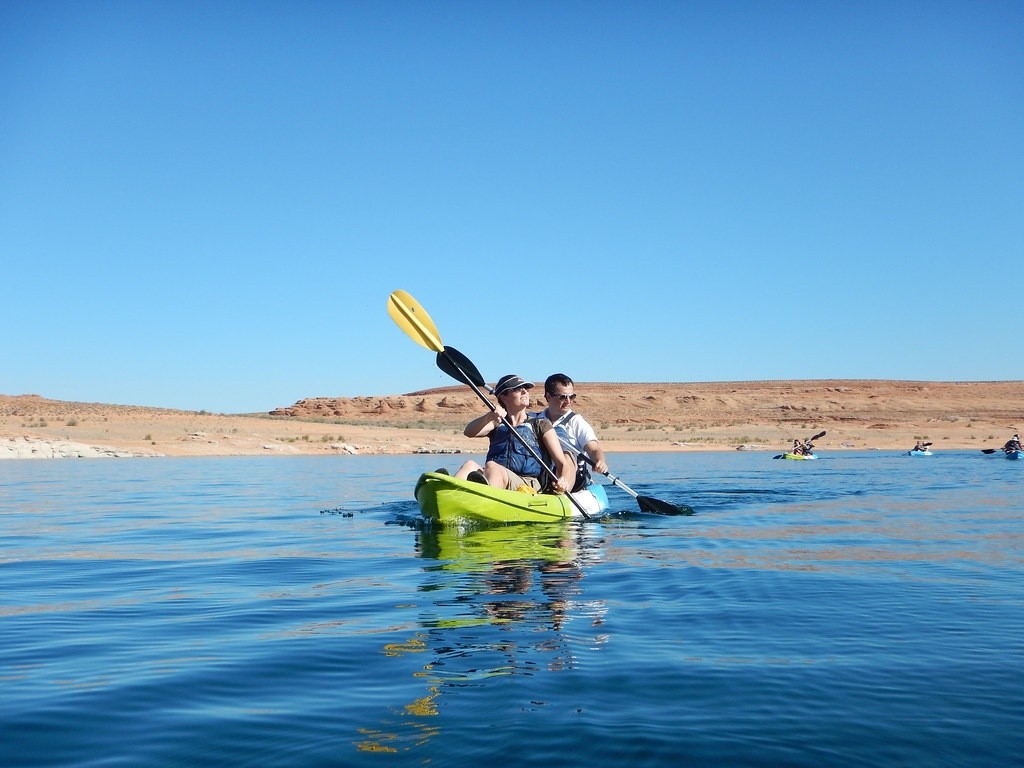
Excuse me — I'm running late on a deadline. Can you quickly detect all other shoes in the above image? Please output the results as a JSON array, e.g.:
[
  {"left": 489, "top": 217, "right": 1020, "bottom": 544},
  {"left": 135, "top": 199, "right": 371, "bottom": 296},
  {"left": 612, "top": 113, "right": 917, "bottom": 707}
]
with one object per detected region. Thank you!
[
  {"left": 434, "top": 468, "right": 450, "bottom": 476},
  {"left": 467, "top": 471, "right": 489, "bottom": 485}
]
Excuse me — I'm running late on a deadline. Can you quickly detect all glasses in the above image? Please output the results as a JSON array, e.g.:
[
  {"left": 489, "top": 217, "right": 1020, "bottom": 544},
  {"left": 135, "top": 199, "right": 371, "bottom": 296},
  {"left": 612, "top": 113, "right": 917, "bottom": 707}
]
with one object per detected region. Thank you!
[
  {"left": 508, "top": 384, "right": 530, "bottom": 393},
  {"left": 550, "top": 394, "right": 576, "bottom": 401}
]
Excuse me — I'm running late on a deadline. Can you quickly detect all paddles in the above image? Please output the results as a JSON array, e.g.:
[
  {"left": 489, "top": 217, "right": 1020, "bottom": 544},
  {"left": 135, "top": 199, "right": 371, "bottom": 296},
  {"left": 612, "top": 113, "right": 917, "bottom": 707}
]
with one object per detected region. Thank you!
[
  {"left": 773, "top": 431, "right": 826, "bottom": 459},
  {"left": 907, "top": 443, "right": 932, "bottom": 452},
  {"left": 981, "top": 447, "right": 1024, "bottom": 454},
  {"left": 436, "top": 345, "right": 680, "bottom": 516},
  {"left": 387, "top": 289, "right": 592, "bottom": 521}
]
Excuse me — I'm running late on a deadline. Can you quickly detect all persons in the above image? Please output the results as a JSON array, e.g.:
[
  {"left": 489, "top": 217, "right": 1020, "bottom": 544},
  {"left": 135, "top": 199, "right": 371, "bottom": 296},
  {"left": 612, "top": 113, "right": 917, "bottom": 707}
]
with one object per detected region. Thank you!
[
  {"left": 525, "top": 374, "right": 608, "bottom": 495},
  {"left": 913, "top": 440, "right": 928, "bottom": 452},
  {"left": 790, "top": 438, "right": 816, "bottom": 456},
  {"left": 1001, "top": 434, "right": 1024, "bottom": 454},
  {"left": 453, "top": 375, "right": 571, "bottom": 494}
]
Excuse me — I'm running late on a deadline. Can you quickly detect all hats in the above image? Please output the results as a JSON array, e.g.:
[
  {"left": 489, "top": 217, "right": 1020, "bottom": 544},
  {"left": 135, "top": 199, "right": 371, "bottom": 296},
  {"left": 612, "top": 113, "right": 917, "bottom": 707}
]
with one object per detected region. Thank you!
[{"left": 495, "top": 375, "right": 535, "bottom": 398}]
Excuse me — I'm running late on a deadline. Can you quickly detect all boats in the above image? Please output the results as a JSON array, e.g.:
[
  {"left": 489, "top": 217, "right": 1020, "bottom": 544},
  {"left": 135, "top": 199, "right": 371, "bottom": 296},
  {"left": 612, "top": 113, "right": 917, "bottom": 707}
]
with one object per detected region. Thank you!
[
  {"left": 782, "top": 453, "right": 819, "bottom": 462},
  {"left": 411, "top": 470, "right": 612, "bottom": 525},
  {"left": 909, "top": 450, "right": 933, "bottom": 456},
  {"left": 1004, "top": 449, "right": 1024, "bottom": 460}
]
